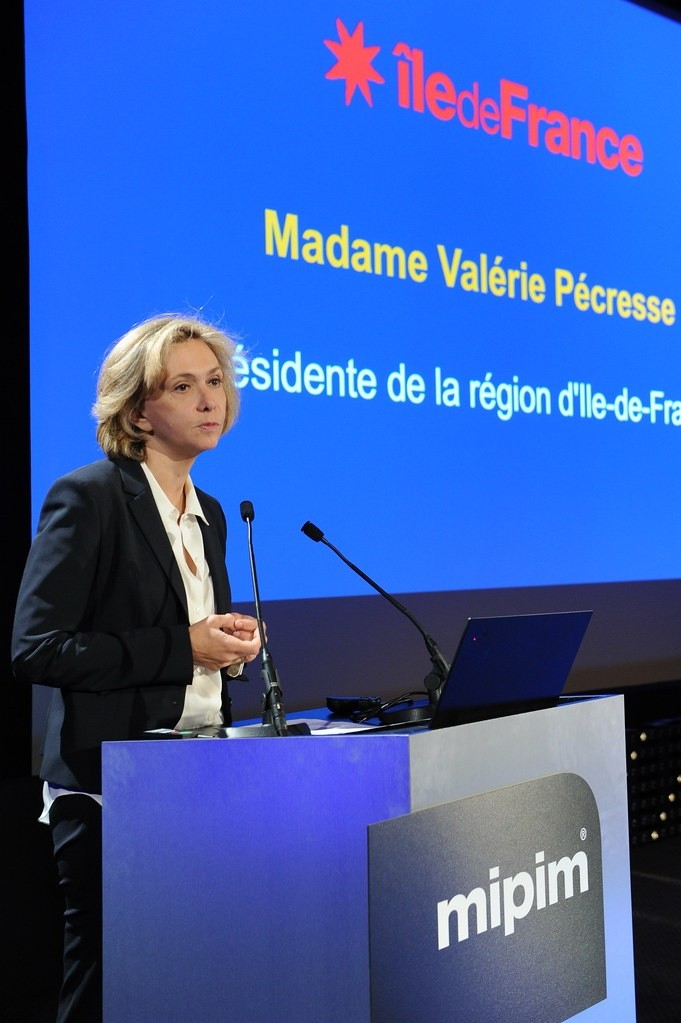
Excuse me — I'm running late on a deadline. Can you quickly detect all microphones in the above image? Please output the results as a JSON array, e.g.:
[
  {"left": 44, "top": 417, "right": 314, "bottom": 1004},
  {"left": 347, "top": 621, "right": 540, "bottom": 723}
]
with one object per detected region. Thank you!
[
  {"left": 240, "top": 500, "right": 312, "bottom": 741},
  {"left": 301, "top": 520, "right": 451, "bottom": 679}
]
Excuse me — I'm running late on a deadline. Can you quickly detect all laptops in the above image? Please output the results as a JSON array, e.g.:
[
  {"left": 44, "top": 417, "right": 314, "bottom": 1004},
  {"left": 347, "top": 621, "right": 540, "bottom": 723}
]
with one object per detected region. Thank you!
[{"left": 428, "top": 610, "right": 595, "bottom": 731}]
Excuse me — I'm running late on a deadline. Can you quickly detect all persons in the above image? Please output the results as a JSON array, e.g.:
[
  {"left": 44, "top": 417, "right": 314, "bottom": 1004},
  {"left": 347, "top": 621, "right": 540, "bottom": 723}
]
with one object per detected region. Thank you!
[{"left": 8, "top": 314, "right": 268, "bottom": 1022}]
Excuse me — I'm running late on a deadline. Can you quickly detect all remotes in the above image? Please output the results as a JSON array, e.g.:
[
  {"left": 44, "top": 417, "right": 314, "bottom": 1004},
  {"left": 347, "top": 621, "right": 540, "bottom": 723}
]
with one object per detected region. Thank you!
[{"left": 324, "top": 695, "right": 379, "bottom": 714}]
